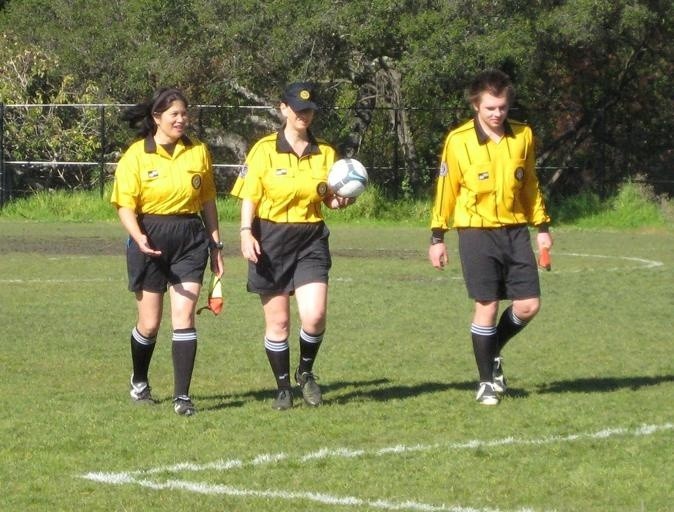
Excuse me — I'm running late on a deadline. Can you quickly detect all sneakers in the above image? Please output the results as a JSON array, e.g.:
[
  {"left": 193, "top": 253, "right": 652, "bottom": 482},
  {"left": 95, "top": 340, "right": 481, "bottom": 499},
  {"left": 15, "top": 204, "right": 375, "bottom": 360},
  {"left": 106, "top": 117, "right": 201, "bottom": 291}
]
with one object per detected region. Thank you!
[
  {"left": 273, "top": 389, "right": 293, "bottom": 411},
  {"left": 475, "top": 353, "right": 507, "bottom": 406},
  {"left": 295, "top": 367, "right": 323, "bottom": 408},
  {"left": 171, "top": 394, "right": 198, "bottom": 418},
  {"left": 128, "top": 372, "right": 157, "bottom": 406}
]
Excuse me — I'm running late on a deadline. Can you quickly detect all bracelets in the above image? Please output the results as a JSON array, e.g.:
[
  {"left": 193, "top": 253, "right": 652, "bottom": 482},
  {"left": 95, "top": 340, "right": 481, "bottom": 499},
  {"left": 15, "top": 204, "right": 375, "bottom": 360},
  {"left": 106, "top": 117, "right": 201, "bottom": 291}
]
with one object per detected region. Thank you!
[
  {"left": 238, "top": 227, "right": 253, "bottom": 231},
  {"left": 209, "top": 242, "right": 223, "bottom": 250},
  {"left": 429, "top": 236, "right": 443, "bottom": 245}
]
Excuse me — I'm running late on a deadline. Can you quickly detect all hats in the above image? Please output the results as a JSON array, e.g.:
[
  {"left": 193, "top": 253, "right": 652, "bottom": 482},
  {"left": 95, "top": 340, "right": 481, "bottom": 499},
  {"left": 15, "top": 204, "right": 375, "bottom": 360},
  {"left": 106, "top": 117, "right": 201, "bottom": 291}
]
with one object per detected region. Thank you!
[{"left": 282, "top": 82, "right": 318, "bottom": 113}]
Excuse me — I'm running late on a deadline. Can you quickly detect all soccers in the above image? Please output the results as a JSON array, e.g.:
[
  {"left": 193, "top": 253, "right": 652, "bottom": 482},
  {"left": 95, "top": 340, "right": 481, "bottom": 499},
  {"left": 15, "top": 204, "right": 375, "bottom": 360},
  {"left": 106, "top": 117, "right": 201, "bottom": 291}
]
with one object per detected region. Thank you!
[{"left": 330, "top": 159, "right": 367, "bottom": 196}]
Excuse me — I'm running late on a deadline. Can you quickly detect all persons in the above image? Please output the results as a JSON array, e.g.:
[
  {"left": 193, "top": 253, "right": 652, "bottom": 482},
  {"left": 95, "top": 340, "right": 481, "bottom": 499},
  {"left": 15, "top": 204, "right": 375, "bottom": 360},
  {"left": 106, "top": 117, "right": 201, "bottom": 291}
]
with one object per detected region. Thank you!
[
  {"left": 428, "top": 67, "right": 553, "bottom": 406},
  {"left": 230, "top": 83, "right": 356, "bottom": 411},
  {"left": 110, "top": 86, "right": 226, "bottom": 416}
]
